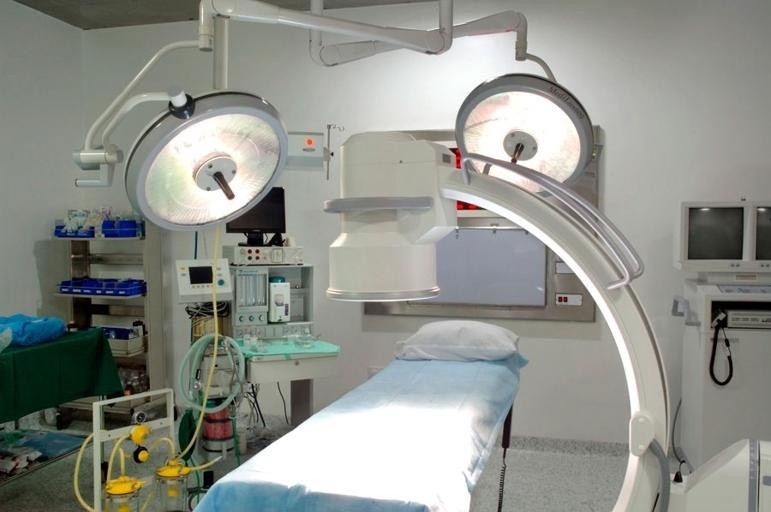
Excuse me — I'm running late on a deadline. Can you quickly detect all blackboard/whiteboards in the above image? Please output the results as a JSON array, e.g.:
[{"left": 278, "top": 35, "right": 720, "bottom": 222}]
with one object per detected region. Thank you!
[{"left": 408, "top": 225, "right": 548, "bottom": 310}]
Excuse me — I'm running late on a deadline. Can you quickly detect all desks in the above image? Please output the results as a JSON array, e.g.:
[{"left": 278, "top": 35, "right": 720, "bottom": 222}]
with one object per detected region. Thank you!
[{"left": 0, "top": 327, "right": 129, "bottom": 486}]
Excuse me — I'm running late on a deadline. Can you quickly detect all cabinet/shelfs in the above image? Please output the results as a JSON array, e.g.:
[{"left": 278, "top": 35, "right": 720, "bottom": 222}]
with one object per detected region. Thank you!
[{"left": 46, "top": 213, "right": 172, "bottom": 433}]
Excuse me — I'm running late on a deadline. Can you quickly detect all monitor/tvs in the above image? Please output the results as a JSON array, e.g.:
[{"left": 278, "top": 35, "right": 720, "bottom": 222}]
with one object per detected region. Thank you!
[
  {"left": 680, "top": 200, "right": 771, "bottom": 285},
  {"left": 226, "top": 187, "right": 286, "bottom": 247}
]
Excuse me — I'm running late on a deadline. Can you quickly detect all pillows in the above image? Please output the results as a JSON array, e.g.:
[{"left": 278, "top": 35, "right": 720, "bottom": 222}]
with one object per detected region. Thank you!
[{"left": 393, "top": 316, "right": 521, "bottom": 363}]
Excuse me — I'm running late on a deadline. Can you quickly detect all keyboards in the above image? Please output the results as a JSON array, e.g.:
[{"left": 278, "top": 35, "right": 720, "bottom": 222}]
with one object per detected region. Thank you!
[{"left": 716, "top": 285, "right": 771, "bottom": 294}]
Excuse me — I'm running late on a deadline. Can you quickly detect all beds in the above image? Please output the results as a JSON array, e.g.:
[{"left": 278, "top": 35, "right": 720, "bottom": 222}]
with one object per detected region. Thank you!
[{"left": 181, "top": 352, "right": 532, "bottom": 511}]
